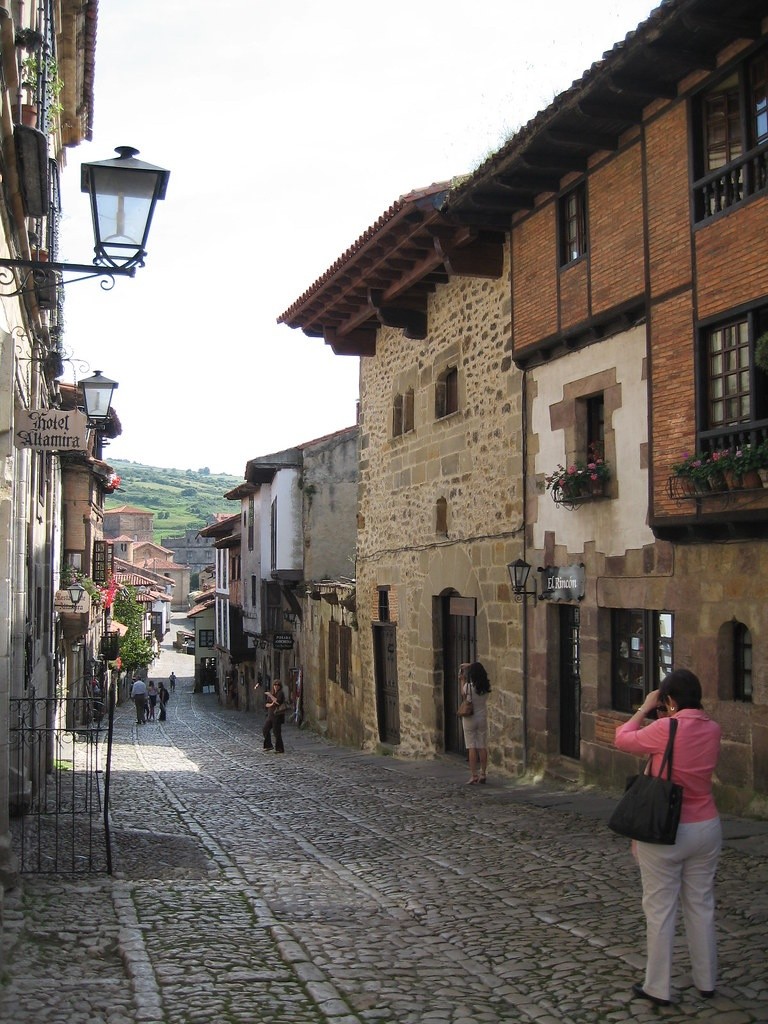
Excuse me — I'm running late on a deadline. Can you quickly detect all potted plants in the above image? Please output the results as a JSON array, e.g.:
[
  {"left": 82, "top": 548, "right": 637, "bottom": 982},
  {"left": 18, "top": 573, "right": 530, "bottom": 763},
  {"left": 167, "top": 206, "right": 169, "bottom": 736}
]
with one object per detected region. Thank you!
[
  {"left": 690, "top": 469, "right": 768, "bottom": 493},
  {"left": 12, "top": 52, "right": 64, "bottom": 146},
  {"left": 31, "top": 246, "right": 48, "bottom": 262}
]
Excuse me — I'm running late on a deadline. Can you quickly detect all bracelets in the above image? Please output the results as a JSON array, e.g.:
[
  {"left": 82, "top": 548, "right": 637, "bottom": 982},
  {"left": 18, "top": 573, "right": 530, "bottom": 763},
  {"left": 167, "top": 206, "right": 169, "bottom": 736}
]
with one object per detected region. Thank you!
[{"left": 638, "top": 708, "right": 647, "bottom": 715}]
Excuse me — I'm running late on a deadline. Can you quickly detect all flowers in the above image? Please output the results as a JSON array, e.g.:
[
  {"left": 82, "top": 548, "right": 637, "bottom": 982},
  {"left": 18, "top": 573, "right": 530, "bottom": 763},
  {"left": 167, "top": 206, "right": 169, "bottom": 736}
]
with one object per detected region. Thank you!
[
  {"left": 671, "top": 438, "right": 768, "bottom": 479},
  {"left": 61, "top": 565, "right": 120, "bottom": 608},
  {"left": 544, "top": 441, "right": 609, "bottom": 488}
]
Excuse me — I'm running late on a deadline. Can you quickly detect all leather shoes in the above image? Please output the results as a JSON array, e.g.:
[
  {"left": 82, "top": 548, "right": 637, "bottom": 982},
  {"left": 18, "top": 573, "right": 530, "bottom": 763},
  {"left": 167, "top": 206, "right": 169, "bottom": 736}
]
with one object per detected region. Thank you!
[
  {"left": 701, "top": 990, "right": 714, "bottom": 998},
  {"left": 633, "top": 983, "right": 669, "bottom": 1007}
]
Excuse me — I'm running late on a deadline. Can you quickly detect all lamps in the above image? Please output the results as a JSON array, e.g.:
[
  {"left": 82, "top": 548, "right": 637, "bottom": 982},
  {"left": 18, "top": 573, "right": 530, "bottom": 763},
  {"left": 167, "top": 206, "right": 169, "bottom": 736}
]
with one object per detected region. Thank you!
[
  {"left": 507, "top": 559, "right": 537, "bottom": 608},
  {"left": 54, "top": 580, "right": 86, "bottom": 610},
  {"left": 252, "top": 635, "right": 266, "bottom": 650},
  {"left": 88, "top": 658, "right": 97, "bottom": 668},
  {"left": 78, "top": 371, "right": 118, "bottom": 430},
  {"left": 71, "top": 636, "right": 85, "bottom": 654},
  {"left": 0, "top": 144, "right": 170, "bottom": 297}
]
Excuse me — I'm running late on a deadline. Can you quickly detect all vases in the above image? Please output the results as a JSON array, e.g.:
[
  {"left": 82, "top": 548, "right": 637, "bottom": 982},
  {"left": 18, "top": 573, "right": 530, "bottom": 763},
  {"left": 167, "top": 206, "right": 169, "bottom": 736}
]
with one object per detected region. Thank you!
[{"left": 561, "top": 480, "right": 605, "bottom": 497}]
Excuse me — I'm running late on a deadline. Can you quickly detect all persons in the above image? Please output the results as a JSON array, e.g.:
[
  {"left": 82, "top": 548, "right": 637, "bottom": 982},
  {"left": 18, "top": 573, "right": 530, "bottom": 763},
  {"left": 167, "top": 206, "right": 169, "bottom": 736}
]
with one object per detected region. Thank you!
[
  {"left": 262, "top": 681, "right": 285, "bottom": 753},
  {"left": 459, "top": 662, "right": 490, "bottom": 784},
  {"left": 131, "top": 675, "right": 168, "bottom": 724},
  {"left": 615, "top": 668, "right": 723, "bottom": 1005},
  {"left": 169, "top": 672, "right": 176, "bottom": 692}
]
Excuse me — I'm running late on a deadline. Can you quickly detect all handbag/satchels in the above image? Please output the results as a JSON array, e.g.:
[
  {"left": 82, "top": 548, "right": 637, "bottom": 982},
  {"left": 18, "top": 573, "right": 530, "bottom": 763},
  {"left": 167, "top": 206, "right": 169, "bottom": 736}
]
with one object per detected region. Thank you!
[
  {"left": 607, "top": 718, "right": 682, "bottom": 846},
  {"left": 156, "top": 695, "right": 161, "bottom": 703},
  {"left": 456, "top": 683, "right": 473, "bottom": 716},
  {"left": 274, "top": 691, "right": 291, "bottom": 715}
]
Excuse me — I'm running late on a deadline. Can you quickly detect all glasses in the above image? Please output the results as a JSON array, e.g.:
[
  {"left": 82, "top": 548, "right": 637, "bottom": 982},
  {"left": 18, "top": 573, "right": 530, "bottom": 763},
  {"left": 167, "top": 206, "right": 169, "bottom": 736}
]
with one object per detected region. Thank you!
[{"left": 273, "top": 683, "right": 277, "bottom": 686}]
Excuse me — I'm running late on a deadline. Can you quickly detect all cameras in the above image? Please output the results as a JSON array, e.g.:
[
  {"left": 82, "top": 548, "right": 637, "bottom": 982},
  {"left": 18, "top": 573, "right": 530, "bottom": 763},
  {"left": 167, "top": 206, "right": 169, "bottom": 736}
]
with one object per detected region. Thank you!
[
  {"left": 460, "top": 668, "right": 465, "bottom": 675},
  {"left": 657, "top": 694, "right": 668, "bottom": 711}
]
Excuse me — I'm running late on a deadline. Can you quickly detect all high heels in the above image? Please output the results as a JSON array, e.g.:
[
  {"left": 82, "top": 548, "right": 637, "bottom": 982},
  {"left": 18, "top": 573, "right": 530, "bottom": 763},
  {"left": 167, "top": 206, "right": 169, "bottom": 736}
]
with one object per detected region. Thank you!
[
  {"left": 466, "top": 778, "right": 478, "bottom": 785},
  {"left": 478, "top": 774, "right": 485, "bottom": 784}
]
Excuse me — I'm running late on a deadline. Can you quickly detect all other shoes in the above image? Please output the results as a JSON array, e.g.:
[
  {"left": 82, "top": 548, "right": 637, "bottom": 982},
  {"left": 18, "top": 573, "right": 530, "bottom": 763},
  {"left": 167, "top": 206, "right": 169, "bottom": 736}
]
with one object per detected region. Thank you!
[
  {"left": 263, "top": 747, "right": 274, "bottom": 751},
  {"left": 272, "top": 750, "right": 284, "bottom": 753}
]
